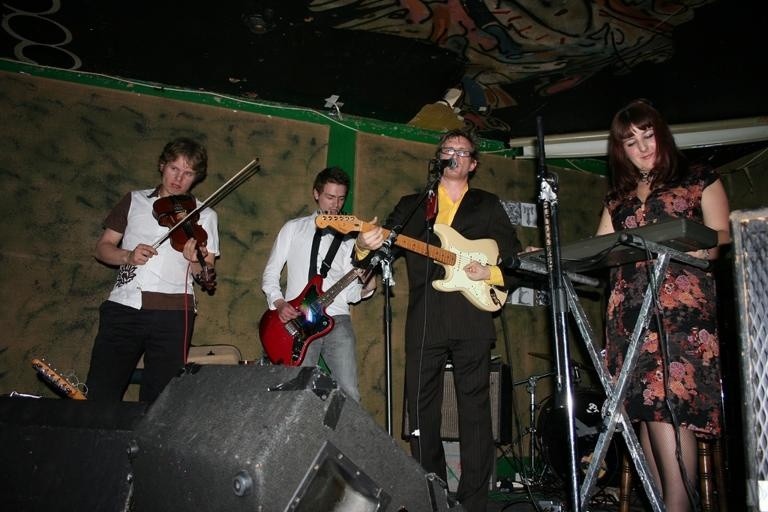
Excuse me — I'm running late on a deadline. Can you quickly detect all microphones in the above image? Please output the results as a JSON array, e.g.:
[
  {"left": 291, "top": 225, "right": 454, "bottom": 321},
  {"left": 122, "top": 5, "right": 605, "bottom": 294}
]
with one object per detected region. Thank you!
[{"left": 431, "top": 158, "right": 456, "bottom": 169}]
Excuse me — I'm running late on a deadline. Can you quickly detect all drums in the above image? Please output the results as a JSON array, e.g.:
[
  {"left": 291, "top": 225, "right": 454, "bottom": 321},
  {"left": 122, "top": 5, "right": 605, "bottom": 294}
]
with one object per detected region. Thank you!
[{"left": 529, "top": 393, "right": 626, "bottom": 487}]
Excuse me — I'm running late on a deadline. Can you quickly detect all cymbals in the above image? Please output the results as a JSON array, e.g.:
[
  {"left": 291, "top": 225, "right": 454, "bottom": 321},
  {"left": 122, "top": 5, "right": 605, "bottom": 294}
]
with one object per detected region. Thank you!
[{"left": 526, "top": 353, "right": 587, "bottom": 371}]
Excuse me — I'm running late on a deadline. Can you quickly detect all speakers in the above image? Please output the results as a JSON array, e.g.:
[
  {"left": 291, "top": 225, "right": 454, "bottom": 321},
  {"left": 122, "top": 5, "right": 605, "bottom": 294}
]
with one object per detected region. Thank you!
[
  {"left": 0, "top": 394, "right": 148, "bottom": 512},
  {"left": 132, "top": 362, "right": 448, "bottom": 512}
]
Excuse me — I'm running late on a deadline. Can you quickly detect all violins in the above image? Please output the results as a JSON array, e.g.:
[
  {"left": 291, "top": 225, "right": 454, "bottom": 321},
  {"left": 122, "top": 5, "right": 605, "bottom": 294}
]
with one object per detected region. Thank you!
[{"left": 153, "top": 195, "right": 217, "bottom": 297}]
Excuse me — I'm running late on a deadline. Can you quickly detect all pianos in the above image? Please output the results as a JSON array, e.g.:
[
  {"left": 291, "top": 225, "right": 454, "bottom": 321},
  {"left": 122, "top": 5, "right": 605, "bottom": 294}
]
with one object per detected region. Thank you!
[{"left": 523, "top": 218, "right": 719, "bottom": 272}]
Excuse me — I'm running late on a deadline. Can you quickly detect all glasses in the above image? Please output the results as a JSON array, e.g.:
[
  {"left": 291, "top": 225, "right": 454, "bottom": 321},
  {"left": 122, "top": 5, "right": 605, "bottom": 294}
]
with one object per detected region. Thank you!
[{"left": 440, "top": 147, "right": 472, "bottom": 157}]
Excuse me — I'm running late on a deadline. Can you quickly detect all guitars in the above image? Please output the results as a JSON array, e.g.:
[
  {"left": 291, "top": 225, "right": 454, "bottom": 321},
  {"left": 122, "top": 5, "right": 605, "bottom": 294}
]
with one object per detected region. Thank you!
[
  {"left": 315, "top": 208, "right": 509, "bottom": 312},
  {"left": 31, "top": 357, "right": 88, "bottom": 400},
  {"left": 259, "top": 266, "right": 366, "bottom": 365}
]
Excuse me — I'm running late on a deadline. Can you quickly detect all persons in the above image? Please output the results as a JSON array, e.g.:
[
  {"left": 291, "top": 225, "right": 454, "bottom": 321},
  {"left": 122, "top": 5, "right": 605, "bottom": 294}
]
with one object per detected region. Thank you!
[
  {"left": 261, "top": 166, "right": 377, "bottom": 403},
  {"left": 517, "top": 101, "right": 730, "bottom": 512},
  {"left": 84, "top": 136, "right": 221, "bottom": 402},
  {"left": 350, "top": 129, "right": 523, "bottom": 511}
]
